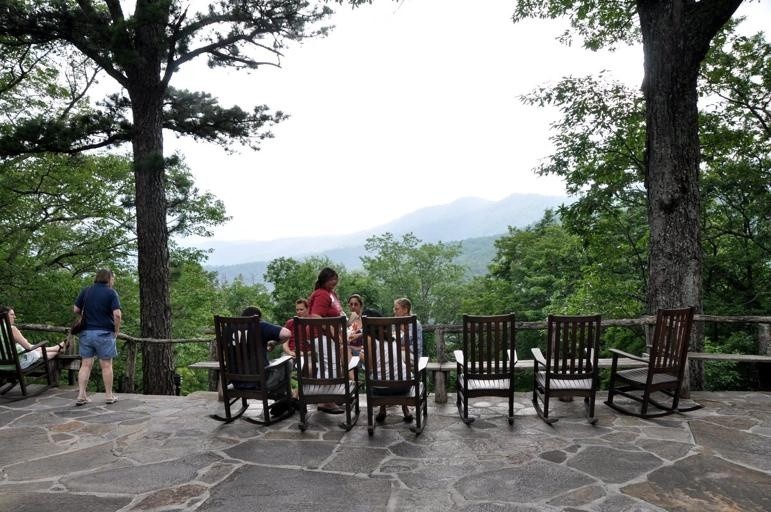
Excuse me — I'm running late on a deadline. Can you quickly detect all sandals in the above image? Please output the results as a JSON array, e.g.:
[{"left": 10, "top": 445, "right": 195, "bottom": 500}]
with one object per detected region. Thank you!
[
  {"left": 106, "top": 394, "right": 119, "bottom": 404},
  {"left": 76, "top": 396, "right": 91, "bottom": 406},
  {"left": 56, "top": 338, "right": 69, "bottom": 354}
]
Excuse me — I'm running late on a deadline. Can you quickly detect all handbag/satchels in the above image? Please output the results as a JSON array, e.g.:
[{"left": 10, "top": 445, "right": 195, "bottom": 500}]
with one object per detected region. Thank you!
[{"left": 71, "top": 319, "right": 82, "bottom": 335}]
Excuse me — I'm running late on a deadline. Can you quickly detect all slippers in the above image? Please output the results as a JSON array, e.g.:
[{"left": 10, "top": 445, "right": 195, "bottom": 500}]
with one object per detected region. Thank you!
[
  {"left": 317, "top": 402, "right": 344, "bottom": 414},
  {"left": 404, "top": 414, "right": 413, "bottom": 422},
  {"left": 375, "top": 414, "right": 385, "bottom": 423}
]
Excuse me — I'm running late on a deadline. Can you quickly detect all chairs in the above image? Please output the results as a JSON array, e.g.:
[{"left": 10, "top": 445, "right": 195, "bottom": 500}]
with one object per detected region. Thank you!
[{"left": 0, "top": 311, "right": 60, "bottom": 404}]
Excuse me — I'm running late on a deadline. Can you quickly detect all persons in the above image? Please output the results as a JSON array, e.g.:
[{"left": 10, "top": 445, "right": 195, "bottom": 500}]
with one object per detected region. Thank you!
[
  {"left": 224, "top": 265, "right": 426, "bottom": 423},
  {"left": 73, "top": 268, "right": 122, "bottom": 407},
  {"left": 0, "top": 305, "right": 70, "bottom": 371}
]
